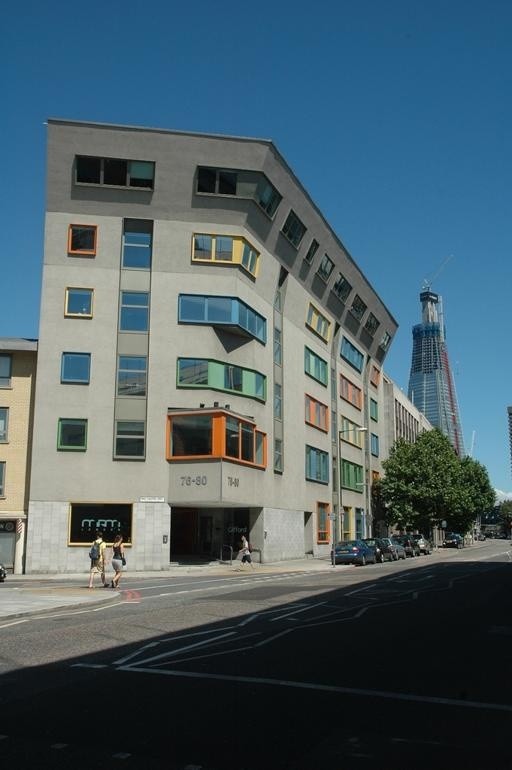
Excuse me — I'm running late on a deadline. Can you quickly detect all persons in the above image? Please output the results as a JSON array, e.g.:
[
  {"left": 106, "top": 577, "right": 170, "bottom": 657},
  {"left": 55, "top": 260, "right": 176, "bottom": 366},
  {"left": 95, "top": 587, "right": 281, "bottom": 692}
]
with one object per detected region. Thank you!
[
  {"left": 88, "top": 531, "right": 110, "bottom": 588},
  {"left": 237, "top": 536, "right": 256, "bottom": 572},
  {"left": 111, "top": 534, "right": 126, "bottom": 588}
]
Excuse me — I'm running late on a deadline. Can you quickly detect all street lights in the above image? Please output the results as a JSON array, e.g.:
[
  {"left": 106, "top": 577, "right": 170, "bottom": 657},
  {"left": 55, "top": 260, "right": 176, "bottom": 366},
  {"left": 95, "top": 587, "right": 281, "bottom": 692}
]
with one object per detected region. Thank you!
[{"left": 339, "top": 427, "right": 368, "bottom": 541}]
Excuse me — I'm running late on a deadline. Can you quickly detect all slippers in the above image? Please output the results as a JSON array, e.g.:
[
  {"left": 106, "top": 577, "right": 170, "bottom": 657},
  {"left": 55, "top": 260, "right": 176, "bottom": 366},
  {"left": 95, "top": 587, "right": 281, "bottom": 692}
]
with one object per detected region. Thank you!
[
  {"left": 89, "top": 586, "right": 95, "bottom": 588},
  {"left": 103, "top": 584, "right": 110, "bottom": 587}
]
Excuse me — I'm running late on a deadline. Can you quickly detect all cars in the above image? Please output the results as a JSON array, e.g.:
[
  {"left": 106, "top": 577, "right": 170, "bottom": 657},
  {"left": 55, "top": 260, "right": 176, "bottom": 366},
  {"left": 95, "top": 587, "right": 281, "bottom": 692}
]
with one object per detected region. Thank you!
[
  {"left": 443, "top": 534, "right": 463, "bottom": 548},
  {"left": 331, "top": 534, "right": 430, "bottom": 566}
]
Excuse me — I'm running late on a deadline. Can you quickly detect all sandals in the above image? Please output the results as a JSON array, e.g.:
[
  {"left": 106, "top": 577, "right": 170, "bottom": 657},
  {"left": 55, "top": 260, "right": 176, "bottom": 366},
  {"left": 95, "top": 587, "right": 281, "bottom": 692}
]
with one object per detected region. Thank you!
[{"left": 112, "top": 580, "right": 120, "bottom": 588}]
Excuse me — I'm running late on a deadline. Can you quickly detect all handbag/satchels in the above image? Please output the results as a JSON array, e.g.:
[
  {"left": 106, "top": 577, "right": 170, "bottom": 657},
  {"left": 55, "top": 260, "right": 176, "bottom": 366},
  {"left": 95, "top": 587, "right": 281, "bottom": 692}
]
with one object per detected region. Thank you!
[{"left": 122, "top": 557, "right": 126, "bottom": 565}]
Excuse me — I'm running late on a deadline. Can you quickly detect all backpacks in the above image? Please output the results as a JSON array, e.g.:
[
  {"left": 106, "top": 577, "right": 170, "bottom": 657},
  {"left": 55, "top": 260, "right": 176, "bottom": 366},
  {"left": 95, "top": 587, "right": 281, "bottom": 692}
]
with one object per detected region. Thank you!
[
  {"left": 89, "top": 542, "right": 101, "bottom": 560},
  {"left": 248, "top": 544, "right": 253, "bottom": 553}
]
